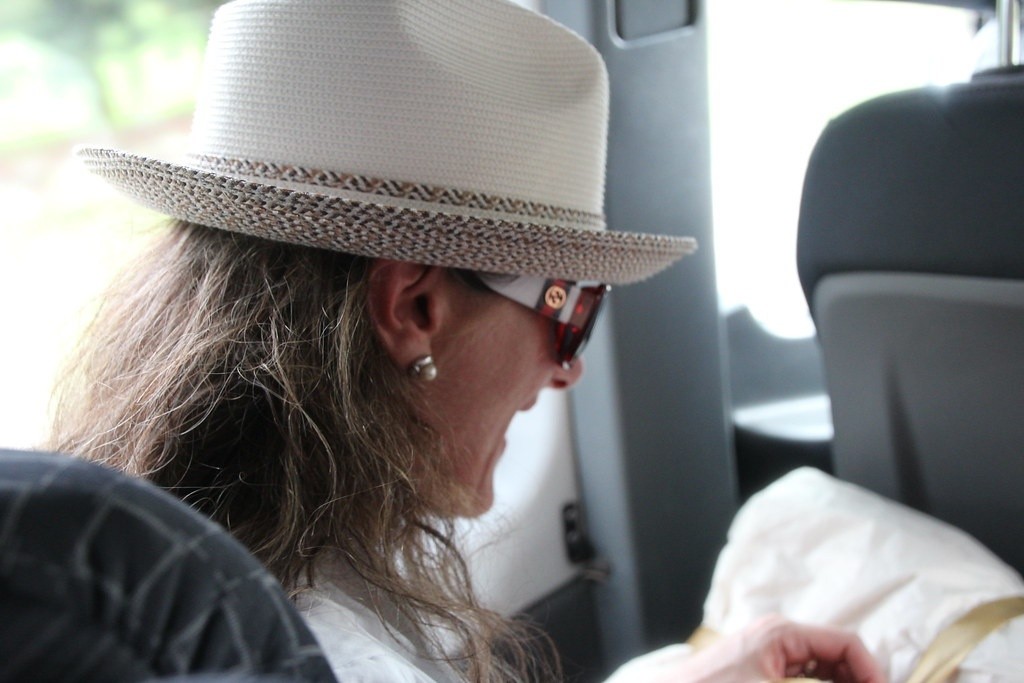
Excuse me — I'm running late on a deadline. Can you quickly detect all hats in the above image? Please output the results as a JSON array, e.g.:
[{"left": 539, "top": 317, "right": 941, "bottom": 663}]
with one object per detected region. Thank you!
[{"left": 73, "top": 0, "right": 700, "bottom": 288}]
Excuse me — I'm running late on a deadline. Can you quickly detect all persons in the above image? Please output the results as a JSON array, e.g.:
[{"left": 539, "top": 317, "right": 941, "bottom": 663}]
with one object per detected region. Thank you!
[
  {"left": 49, "top": 1, "right": 892, "bottom": 683},
  {"left": 0, "top": 447, "right": 342, "bottom": 683}
]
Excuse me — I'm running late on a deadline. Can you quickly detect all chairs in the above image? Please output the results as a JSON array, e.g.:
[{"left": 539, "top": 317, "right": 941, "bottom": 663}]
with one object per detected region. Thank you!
[
  {"left": 1, "top": 447, "right": 337, "bottom": 682},
  {"left": 798, "top": 79, "right": 1024, "bottom": 585}
]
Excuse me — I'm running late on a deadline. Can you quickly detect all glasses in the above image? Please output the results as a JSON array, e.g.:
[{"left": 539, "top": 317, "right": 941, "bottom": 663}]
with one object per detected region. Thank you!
[{"left": 473, "top": 271, "right": 606, "bottom": 365}]
